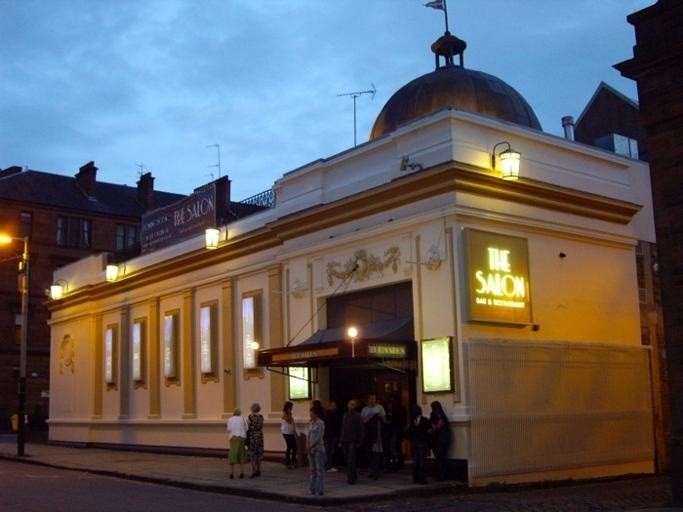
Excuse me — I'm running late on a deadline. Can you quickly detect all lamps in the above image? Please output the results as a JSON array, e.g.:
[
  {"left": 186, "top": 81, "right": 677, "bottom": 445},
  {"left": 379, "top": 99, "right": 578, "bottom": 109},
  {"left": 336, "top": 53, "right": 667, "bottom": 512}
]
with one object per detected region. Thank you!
[
  {"left": 106, "top": 260, "right": 126, "bottom": 283},
  {"left": 49, "top": 279, "right": 68, "bottom": 301},
  {"left": 492, "top": 141, "right": 520, "bottom": 180},
  {"left": 348, "top": 327, "right": 358, "bottom": 358},
  {"left": 251, "top": 342, "right": 259, "bottom": 352},
  {"left": 205, "top": 218, "right": 228, "bottom": 249}
]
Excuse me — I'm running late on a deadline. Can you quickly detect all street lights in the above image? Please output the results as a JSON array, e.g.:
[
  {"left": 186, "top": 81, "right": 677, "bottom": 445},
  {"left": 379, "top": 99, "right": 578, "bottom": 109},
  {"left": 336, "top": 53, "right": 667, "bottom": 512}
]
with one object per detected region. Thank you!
[{"left": 0, "top": 232, "right": 33, "bottom": 460}]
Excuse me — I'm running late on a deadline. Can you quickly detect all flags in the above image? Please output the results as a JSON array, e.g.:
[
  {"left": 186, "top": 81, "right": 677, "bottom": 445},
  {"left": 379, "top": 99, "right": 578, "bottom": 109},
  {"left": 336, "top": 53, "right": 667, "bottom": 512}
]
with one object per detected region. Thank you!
[{"left": 420, "top": 0, "right": 445, "bottom": 14}]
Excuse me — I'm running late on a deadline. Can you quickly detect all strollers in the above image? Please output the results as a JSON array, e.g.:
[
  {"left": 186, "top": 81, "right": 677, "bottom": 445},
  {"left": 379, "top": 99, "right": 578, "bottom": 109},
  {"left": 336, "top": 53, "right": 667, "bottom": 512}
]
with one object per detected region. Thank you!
[{"left": 295, "top": 431, "right": 307, "bottom": 447}]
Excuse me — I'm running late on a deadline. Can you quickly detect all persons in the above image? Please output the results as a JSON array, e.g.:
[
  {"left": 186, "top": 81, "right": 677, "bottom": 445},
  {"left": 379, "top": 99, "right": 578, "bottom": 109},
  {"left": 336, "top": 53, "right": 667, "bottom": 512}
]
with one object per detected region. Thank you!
[
  {"left": 279, "top": 390, "right": 452, "bottom": 484},
  {"left": 244, "top": 402, "right": 263, "bottom": 478},
  {"left": 303, "top": 405, "right": 327, "bottom": 495},
  {"left": 226, "top": 407, "right": 245, "bottom": 479}
]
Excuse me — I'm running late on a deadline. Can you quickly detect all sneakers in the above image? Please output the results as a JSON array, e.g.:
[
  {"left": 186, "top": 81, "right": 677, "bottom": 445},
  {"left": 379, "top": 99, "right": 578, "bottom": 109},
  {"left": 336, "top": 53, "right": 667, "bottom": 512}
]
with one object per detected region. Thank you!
[{"left": 326, "top": 467, "right": 339, "bottom": 472}]
